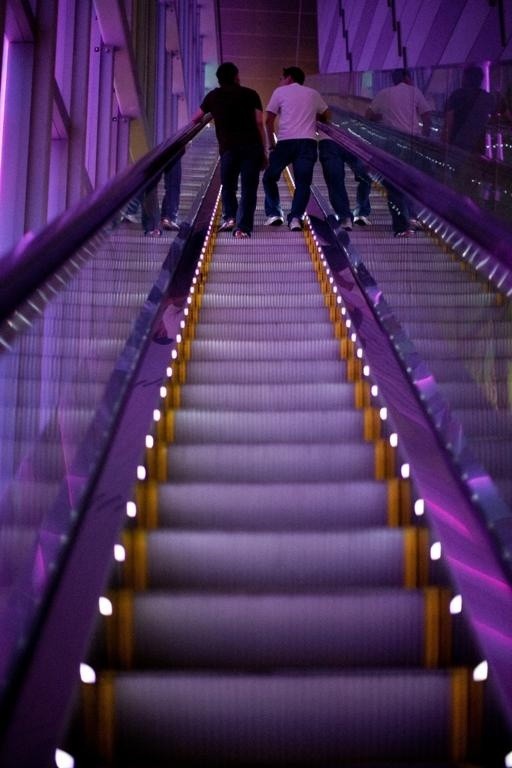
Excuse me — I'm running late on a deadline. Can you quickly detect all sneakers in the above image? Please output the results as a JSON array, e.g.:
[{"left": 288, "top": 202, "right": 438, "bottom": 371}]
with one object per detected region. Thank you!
[
  {"left": 288, "top": 216, "right": 302, "bottom": 231},
  {"left": 162, "top": 218, "right": 181, "bottom": 231},
  {"left": 339, "top": 216, "right": 353, "bottom": 231},
  {"left": 234, "top": 230, "right": 250, "bottom": 238},
  {"left": 264, "top": 216, "right": 284, "bottom": 226},
  {"left": 146, "top": 230, "right": 161, "bottom": 237},
  {"left": 217, "top": 218, "right": 235, "bottom": 232},
  {"left": 395, "top": 230, "right": 416, "bottom": 237},
  {"left": 354, "top": 216, "right": 372, "bottom": 226}
]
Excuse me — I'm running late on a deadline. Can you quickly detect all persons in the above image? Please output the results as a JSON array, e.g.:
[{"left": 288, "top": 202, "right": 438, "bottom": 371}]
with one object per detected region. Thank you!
[
  {"left": 140, "top": 148, "right": 188, "bottom": 240},
  {"left": 367, "top": 66, "right": 430, "bottom": 237},
  {"left": 319, "top": 127, "right": 372, "bottom": 231},
  {"left": 196, "top": 62, "right": 268, "bottom": 239},
  {"left": 264, "top": 64, "right": 330, "bottom": 232},
  {"left": 442, "top": 66, "right": 493, "bottom": 153}
]
